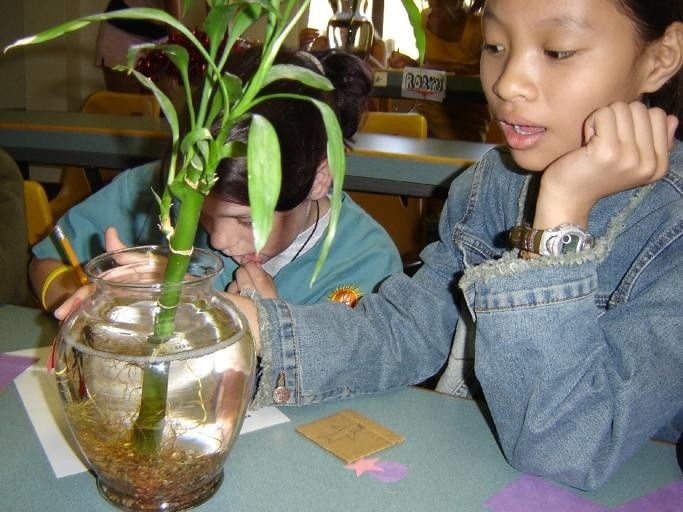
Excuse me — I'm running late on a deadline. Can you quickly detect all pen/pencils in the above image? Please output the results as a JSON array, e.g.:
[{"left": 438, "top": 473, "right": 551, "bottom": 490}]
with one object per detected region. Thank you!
[{"left": 53, "top": 224, "right": 88, "bottom": 285}]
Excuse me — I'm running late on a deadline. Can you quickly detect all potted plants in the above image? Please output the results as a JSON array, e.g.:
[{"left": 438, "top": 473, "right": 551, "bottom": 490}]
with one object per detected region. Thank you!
[{"left": 3, "top": 0, "right": 429, "bottom": 512}]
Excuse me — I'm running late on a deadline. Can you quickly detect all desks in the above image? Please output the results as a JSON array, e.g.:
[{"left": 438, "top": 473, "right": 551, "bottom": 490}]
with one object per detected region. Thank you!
[
  {"left": 0, "top": 132, "right": 509, "bottom": 196},
  {"left": 0, "top": 301, "right": 682, "bottom": 512}
]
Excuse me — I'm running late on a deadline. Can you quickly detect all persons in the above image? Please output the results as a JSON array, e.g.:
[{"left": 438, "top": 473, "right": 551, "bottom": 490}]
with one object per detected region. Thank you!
[
  {"left": 28, "top": 45, "right": 403, "bottom": 314},
  {"left": 53, "top": 0, "right": 683, "bottom": 492},
  {"left": 300, "top": 28, "right": 387, "bottom": 69},
  {"left": 95, "top": 0, "right": 183, "bottom": 94}
]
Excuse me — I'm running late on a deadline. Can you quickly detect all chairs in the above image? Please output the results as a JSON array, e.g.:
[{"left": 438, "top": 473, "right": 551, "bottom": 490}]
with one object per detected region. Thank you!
[
  {"left": 23, "top": 180, "right": 55, "bottom": 247},
  {"left": 48, "top": 92, "right": 162, "bottom": 229},
  {"left": 341, "top": 109, "right": 427, "bottom": 254}
]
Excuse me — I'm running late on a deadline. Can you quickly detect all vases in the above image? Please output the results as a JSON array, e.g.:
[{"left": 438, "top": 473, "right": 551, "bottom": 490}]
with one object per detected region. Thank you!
[{"left": 326, "top": 0, "right": 373, "bottom": 60}]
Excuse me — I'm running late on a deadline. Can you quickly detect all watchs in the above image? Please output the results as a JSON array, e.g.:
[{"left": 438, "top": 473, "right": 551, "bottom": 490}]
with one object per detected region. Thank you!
[{"left": 507, "top": 225, "right": 593, "bottom": 260}]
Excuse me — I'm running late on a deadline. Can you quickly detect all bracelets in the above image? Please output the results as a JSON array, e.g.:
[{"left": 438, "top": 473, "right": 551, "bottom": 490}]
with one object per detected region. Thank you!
[{"left": 42, "top": 266, "right": 75, "bottom": 312}]
